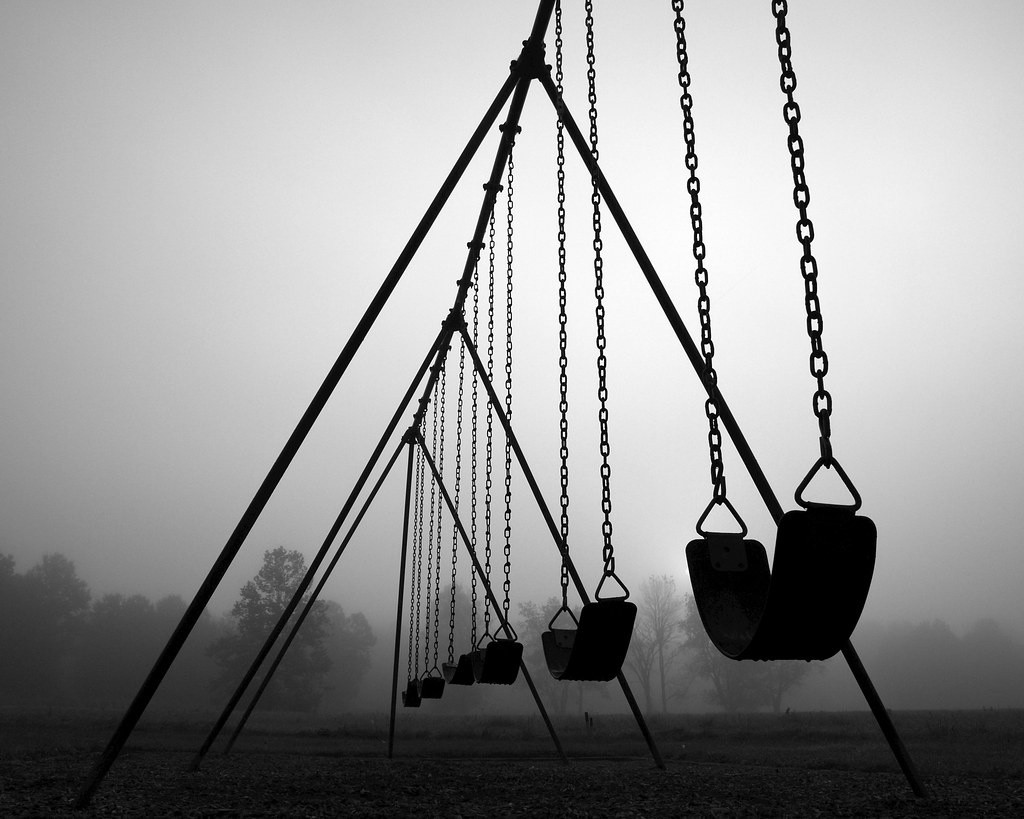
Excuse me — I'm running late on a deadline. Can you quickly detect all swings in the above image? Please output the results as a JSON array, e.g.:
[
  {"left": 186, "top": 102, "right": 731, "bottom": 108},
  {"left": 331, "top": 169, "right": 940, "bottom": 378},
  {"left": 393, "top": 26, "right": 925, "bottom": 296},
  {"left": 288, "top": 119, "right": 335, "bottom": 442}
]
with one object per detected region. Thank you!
[
  {"left": 541, "top": 0, "right": 638, "bottom": 684},
  {"left": 399, "top": 151, "right": 523, "bottom": 709},
  {"left": 667, "top": 1, "right": 878, "bottom": 663}
]
